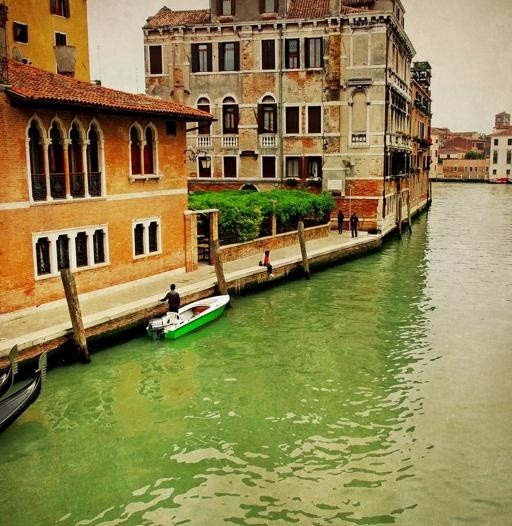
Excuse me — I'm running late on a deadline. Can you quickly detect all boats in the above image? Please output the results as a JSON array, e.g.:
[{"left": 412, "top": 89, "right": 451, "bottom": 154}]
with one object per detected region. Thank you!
[
  {"left": 0, "top": 344, "right": 19, "bottom": 399},
  {"left": 144, "top": 293, "right": 231, "bottom": 342},
  {"left": 0, "top": 350, "right": 49, "bottom": 436}
]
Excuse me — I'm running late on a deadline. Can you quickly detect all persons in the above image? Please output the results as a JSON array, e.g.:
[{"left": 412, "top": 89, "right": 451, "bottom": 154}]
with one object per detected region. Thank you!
[
  {"left": 263, "top": 250, "right": 274, "bottom": 277},
  {"left": 337, "top": 208, "right": 344, "bottom": 234},
  {"left": 350, "top": 213, "right": 358, "bottom": 238},
  {"left": 160, "top": 285, "right": 180, "bottom": 319}
]
